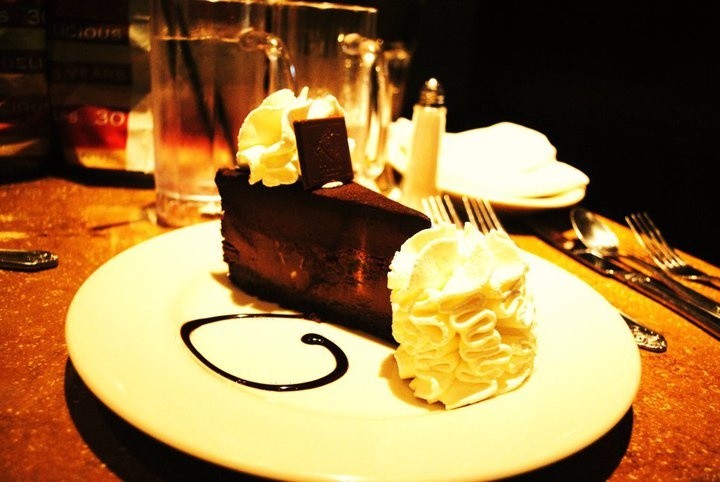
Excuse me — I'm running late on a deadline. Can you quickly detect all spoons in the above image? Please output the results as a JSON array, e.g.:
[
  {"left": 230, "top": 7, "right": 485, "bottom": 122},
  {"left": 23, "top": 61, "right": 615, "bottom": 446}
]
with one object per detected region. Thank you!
[{"left": 570, "top": 207, "right": 720, "bottom": 319}]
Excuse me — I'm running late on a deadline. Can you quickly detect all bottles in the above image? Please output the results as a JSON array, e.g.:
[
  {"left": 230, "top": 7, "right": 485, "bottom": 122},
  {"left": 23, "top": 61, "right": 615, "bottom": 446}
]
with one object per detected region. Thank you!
[{"left": 400, "top": 77, "right": 447, "bottom": 209}]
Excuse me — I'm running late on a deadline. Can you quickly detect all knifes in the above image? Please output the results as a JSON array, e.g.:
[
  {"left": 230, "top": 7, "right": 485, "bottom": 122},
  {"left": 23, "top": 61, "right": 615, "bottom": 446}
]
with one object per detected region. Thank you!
[{"left": 526, "top": 222, "right": 720, "bottom": 341}]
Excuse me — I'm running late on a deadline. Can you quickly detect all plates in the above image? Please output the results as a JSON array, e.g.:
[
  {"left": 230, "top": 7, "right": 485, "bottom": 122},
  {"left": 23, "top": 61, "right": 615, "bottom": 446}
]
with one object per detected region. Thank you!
[
  {"left": 64, "top": 219, "right": 641, "bottom": 481},
  {"left": 387, "top": 154, "right": 586, "bottom": 212}
]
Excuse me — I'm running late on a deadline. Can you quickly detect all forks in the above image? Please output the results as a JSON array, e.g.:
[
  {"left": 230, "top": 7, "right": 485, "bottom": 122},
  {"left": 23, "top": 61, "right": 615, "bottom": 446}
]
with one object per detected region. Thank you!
[
  {"left": 421, "top": 194, "right": 460, "bottom": 226},
  {"left": 462, "top": 197, "right": 668, "bottom": 351},
  {"left": 626, "top": 212, "right": 720, "bottom": 289}
]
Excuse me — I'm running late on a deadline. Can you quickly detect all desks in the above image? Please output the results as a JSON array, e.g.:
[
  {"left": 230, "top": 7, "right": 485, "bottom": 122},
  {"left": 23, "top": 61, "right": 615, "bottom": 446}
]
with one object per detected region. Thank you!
[{"left": 0, "top": 73, "right": 720, "bottom": 482}]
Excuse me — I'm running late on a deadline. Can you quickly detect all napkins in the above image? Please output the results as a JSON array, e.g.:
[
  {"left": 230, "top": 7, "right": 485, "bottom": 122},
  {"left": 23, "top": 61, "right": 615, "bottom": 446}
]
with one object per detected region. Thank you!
[{"left": 384, "top": 116, "right": 590, "bottom": 201}]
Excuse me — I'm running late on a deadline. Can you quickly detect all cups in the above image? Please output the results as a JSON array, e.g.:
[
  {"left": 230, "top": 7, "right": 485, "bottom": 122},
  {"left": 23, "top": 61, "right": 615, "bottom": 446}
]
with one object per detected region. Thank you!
[
  {"left": 269, "top": 0, "right": 392, "bottom": 181},
  {"left": 149, "top": 0, "right": 296, "bottom": 228}
]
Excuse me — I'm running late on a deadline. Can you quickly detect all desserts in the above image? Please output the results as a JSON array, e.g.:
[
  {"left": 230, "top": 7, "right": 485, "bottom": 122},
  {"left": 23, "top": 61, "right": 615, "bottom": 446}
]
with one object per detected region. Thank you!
[{"left": 216, "top": 85, "right": 538, "bottom": 408}]
